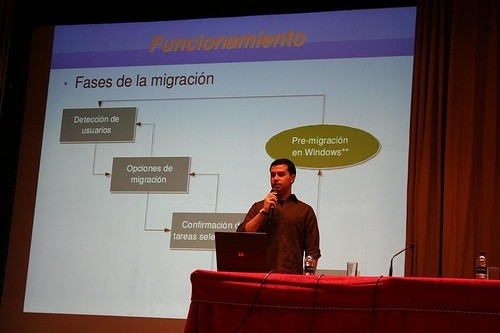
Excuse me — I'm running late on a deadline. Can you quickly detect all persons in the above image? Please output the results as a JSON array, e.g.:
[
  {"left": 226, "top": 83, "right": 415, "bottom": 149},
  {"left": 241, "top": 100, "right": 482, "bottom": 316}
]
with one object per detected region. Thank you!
[{"left": 236, "top": 158, "right": 321, "bottom": 275}]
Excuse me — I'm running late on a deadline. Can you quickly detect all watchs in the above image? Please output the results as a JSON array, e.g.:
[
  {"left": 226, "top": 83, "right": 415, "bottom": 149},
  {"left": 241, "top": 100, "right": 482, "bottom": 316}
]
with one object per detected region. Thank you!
[{"left": 258, "top": 209, "right": 270, "bottom": 215}]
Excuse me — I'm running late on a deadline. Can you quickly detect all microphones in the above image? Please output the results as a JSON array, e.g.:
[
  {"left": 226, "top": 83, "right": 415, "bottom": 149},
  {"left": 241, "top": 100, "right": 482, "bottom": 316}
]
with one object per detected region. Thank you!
[
  {"left": 389, "top": 243, "right": 415, "bottom": 277},
  {"left": 269, "top": 188, "right": 278, "bottom": 220}
]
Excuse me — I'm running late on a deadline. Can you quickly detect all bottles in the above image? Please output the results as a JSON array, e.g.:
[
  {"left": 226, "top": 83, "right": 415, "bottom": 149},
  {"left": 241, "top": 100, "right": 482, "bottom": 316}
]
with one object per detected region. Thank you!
[
  {"left": 305, "top": 250, "right": 314, "bottom": 275},
  {"left": 476, "top": 251, "right": 487, "bottom": 279}
]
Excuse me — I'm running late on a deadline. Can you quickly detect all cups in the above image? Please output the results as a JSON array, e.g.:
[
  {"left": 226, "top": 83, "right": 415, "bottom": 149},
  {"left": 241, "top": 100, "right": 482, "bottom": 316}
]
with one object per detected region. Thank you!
[{"left": 347, "top": 262, "right": 358, "bottom": 276}]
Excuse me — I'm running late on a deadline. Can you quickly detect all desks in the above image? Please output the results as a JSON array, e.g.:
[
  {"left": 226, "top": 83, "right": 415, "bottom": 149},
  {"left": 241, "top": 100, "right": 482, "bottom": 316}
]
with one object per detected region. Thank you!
[{"left": 183, "top": 269, "right": 500, "bottom": 333}]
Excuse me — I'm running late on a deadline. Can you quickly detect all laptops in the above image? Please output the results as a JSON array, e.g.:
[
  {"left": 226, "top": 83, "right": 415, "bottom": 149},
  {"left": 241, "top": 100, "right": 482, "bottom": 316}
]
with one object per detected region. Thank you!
[{"left": 215, "top": 232, "right": 278, "bottom": 273}]
